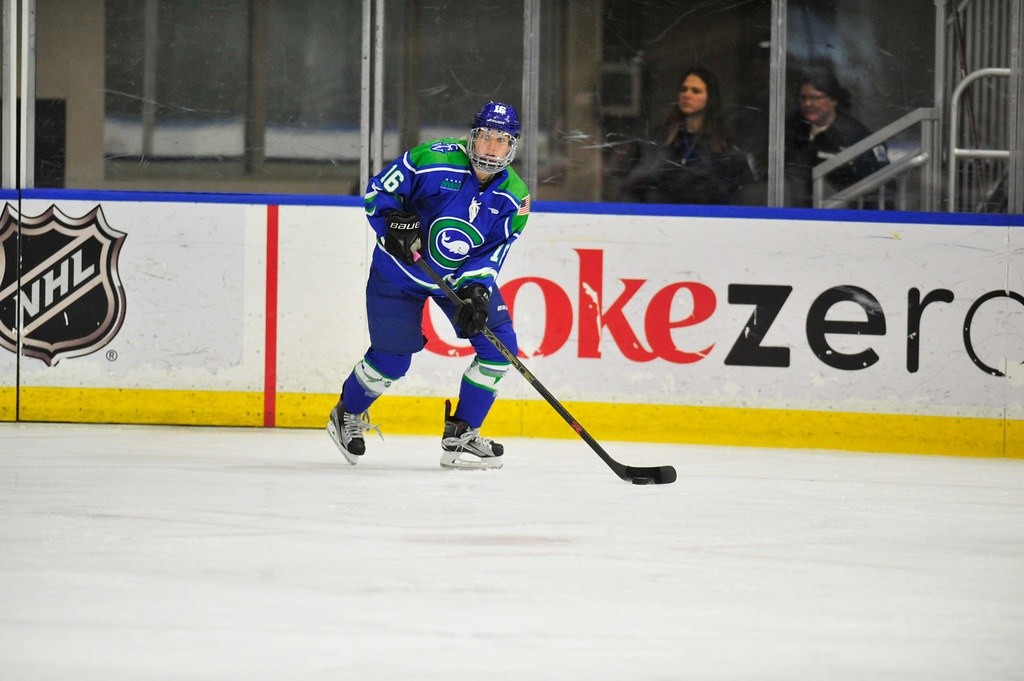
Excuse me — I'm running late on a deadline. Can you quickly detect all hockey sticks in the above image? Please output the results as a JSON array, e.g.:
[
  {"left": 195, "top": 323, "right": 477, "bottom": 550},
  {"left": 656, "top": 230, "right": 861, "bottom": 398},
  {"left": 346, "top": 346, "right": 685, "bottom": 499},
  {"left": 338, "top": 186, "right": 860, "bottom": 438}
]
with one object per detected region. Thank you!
[{"left": 411, "top": 248, "right": 677, "bottom": 484}]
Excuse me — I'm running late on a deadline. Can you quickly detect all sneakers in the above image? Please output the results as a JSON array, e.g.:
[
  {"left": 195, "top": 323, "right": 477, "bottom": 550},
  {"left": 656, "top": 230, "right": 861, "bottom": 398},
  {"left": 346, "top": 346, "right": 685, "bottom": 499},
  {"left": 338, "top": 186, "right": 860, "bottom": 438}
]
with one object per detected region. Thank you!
[
  {"left": 326, "top": 380, "right": 384, "bottom": 466},
  {"left": 440, "top": 399, "right": 504, "bottom": 470}
]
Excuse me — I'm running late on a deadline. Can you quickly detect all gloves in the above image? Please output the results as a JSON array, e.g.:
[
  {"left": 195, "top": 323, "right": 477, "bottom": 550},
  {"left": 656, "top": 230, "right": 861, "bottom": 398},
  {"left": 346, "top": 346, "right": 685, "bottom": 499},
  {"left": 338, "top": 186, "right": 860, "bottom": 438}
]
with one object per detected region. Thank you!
[
  {"left": 384, "top": 214, "right": 421, "bottom": 268},
  {"left": 454, "top": 283, "right": 490, "bottom": 339}
]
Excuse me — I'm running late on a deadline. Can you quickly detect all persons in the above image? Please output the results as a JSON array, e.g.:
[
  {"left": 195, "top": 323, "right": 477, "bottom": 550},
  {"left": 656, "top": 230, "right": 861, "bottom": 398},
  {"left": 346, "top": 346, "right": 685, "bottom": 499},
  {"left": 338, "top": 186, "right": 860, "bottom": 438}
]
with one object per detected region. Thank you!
[
  {"left": 784, "top": 66, "right": 896, "bottom": 210},
  {"left": 650, "top": 67, "right": 748, "bottom": 205},
  {"left": 327, "top": 99, "right": 530, "bottom": 471}
]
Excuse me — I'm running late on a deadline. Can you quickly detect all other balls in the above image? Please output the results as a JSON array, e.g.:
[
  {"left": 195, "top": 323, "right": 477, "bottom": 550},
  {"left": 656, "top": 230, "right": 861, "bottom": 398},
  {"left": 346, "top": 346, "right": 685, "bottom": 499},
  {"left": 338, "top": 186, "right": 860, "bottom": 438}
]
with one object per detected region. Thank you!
[{"left": 632, "top": 476, "right": 655, "bottom": 486}]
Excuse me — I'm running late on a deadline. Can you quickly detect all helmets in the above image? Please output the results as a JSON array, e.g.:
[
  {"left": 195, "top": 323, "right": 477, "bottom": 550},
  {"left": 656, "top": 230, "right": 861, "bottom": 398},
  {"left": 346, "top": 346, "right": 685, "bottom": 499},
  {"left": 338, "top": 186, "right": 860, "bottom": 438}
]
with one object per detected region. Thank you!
[{"left": 467, "top": 100, "right": 521, "bottom": 175}]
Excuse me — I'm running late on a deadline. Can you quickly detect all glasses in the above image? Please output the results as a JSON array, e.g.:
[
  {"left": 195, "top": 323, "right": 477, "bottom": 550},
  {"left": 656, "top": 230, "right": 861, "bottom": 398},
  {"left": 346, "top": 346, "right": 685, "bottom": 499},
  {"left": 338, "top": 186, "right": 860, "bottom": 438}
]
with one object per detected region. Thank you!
[{"left": 798, "top": 93, "right": 827, "bottom": 103}]
